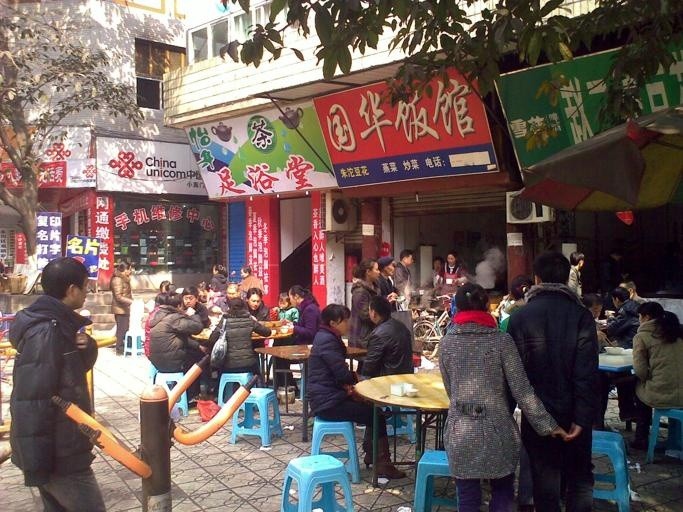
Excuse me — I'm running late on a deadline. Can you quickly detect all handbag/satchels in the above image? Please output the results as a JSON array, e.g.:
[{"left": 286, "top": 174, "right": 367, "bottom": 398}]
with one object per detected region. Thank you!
[
  {"left": 390, "top": 301, "right": 413, "bottom": 334},
  {"left": 210, "top": 331, "right": 228, "bottom": 367}
]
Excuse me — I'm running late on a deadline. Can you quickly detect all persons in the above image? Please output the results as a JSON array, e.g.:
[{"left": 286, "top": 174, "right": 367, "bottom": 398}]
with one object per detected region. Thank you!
[
  {"left": 144, "top": 263, "right": 322, "bottom": 409},
  {"left": 109, "top": 260, "right": 134, "bottom": 356},
  {"left": 8, "top": 257, "right": 106, "bottom": 512},
  {"left": 350, "top": 251, "right": 424, "bottom": 422},
  {"left": 506, "top": 251, "right": 598, "bottom": 512},
  {"left": 565, "top": 251, "right": 648, "bottom": 427},
  {"left": 308, "top": 304, "right": 406, "bottom": 479},
  {"left": 434, "top": 253, "right": 533, "bottom": 328},
  {"left": 629, "top": 301, "right": 683, "bottom": 452},
  {"left": 438, "top": 282, "right": 567, "bottom": 512}
]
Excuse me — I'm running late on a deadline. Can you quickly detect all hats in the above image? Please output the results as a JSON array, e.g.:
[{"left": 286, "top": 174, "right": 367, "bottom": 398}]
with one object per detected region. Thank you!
[{"left": 377, "top": 256, "right": 394, "bottom": 270}]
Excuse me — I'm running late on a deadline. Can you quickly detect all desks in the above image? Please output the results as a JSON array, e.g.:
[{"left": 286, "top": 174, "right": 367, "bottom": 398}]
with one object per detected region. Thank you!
[
  {"left": 354, "top": 373, "right": 450, "bottom": 489},
  {"left": 253, "top": 345, "right": 367, "bottom": 442}
]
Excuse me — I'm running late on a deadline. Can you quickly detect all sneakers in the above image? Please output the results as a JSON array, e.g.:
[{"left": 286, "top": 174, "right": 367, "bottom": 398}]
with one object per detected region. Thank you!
[
  {"left": 629, "top": 440, "right": 648, "bottom": 451},
  {"left": 198, "top": 392, "right": 213, "bottom": 400}
]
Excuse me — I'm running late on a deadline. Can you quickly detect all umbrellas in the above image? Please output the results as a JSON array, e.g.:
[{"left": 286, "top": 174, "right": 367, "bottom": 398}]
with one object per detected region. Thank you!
[{"left": 508, "top": 106, "right": 683, "bottom": 212}]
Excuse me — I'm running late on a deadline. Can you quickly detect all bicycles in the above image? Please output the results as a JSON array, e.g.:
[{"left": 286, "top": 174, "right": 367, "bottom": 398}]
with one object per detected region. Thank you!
[{"left": 411, "top": 294, "right": 453, "bottom": 360}]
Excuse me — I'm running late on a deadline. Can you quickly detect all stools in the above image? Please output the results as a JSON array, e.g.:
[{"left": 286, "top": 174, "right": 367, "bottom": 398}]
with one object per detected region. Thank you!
[
  {"left": 124, "top": 320, "right": 295, "bottom": 447},
  {"left": 413, "top": 450, "right": 460, "bottom": 512},
  {"left": 647, "top": 409, "right": 683, "bottom": 463},
  {"left": 591, "top": 431, "right": 630, "bottom": 512},
  {"left": 311, "top": 416, "right": 360, "bottom": 483},
  {"left": 377, "top": 404, "right": 416, "bottom": 444},
  {"left": 281, "top": 455, "right": 353, "bottom": 511},
  {"left": 299, "top": 363, "right": 311, "bottom": 401}
]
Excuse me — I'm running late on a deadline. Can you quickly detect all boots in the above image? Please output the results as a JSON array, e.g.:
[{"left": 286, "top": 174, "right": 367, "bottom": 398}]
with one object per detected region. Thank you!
[{"left": 364, "top": 427, "right": 406, "bottom": 478}]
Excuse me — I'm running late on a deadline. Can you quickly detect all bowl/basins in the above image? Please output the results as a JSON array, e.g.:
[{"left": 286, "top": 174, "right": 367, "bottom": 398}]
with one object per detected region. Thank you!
[
  {"left": 605, "top": 347, "right": 624, "bottom": 355},
  {"left": 281, "top": 329, "right": 288, "bottom": 333},
  {"left": 272, "top": 330, "right": 276, "bottom": 335},
  {"left": 407, "top": 389, "right": 417, "bottom": 397}
]
